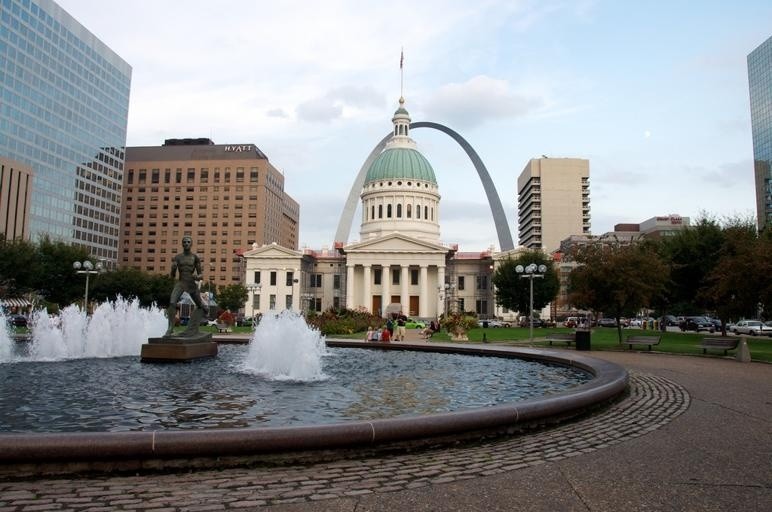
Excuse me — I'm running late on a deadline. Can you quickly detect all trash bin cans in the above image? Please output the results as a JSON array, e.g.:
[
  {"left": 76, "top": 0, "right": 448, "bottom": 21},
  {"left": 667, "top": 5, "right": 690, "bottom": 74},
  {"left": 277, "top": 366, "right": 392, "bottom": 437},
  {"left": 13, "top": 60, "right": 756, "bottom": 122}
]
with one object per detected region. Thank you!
[
  {"left": 483, "top": 321, "right": 488, "bottom": 328},
  {"left": 576, "top": 331, "right": 591, "bottom": 351}
]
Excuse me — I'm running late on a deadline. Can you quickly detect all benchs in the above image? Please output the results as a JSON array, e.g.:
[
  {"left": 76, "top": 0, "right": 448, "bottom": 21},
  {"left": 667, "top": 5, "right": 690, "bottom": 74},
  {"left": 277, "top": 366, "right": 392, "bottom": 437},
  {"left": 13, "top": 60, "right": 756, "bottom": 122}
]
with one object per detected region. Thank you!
[
  {"left": 545, "top": 333, "right": 576, "bottom": 346},
  {"left": 698, "top": 338, "right": 741, "bottom": 356},
  {"left": 623, "top": 335, "right": 661, "bottom": 351}
]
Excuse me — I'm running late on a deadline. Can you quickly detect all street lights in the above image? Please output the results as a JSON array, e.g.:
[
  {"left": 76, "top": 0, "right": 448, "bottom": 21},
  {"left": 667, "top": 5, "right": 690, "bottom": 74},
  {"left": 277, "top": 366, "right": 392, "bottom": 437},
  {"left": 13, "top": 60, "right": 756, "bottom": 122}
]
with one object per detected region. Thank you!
[
  {"left": 70, "top": 260, "right": 108, "bottom": 314},
  {"left": 292, "top": 279, "right": 299, "bottom": 304},
  {"left": 198, "top": 279, "right": 204, "bottom": 290},
  {"left": 244, "top": 282, "right": 264, "bottom": 331},
  {"left": 513, "top": 262, "right": 549, "bottom": 348},
  {"left": 435, "top": 282, "right": 457, "bottom": 317},
  {"left": 206, "top": 278, "right": 213, "bottom": 317}
]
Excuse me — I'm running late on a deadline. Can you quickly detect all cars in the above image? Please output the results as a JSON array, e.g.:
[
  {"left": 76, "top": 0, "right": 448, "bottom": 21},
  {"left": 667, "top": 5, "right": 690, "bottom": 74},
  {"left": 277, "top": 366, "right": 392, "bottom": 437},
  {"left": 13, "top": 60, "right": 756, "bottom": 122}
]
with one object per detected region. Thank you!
[
  {"left": 4, "top": 312, "right": 29, "bottom": 328},
  {"left": 389, "top": 312, "right": 442, "bottom": 330},
  {"left": 174, "top": 315, "right": 257, "bottom": 327},
  {"left": 476, "top": 313, "right": 772, "bottom": 338}
]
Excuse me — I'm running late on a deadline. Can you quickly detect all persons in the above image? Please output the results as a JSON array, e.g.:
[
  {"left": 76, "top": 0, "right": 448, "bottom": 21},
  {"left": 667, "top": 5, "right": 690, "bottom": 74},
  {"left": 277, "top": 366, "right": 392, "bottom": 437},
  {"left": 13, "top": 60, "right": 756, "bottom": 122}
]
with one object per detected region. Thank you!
[
  {"left": 418, "top": 320, "right": 440, "bottom": 339},
  {"left": 164, "top": 237, "right": 210, "bottom": 338},
  {"left": 366, "top": 310, "right": 407, "bottom": 342},
  {"left": 577, "top": 315, "right": 586, "bottom": 328}
]
